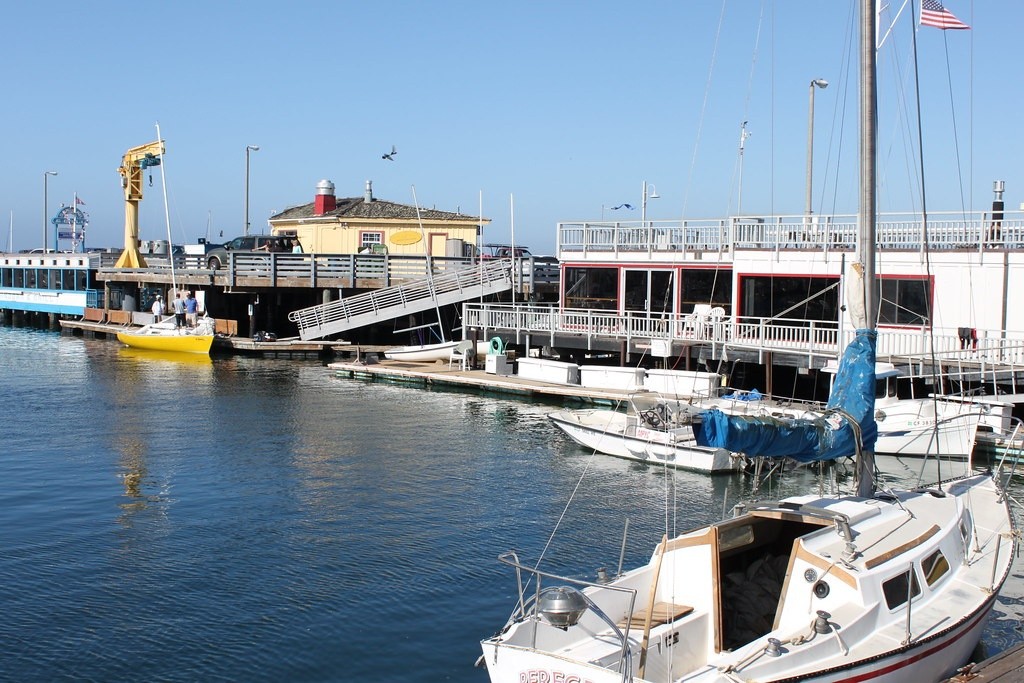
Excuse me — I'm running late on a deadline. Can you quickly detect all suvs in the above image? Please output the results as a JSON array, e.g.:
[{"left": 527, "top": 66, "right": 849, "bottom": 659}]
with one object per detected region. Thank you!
[{"left": 205, "top": 233, "right": 304, "bottom": 270}]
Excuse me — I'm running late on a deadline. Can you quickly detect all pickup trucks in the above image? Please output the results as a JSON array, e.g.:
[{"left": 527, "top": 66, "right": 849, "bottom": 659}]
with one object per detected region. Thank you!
[{"left": 476, "top": 246, "right": 533, "bottom": 263}]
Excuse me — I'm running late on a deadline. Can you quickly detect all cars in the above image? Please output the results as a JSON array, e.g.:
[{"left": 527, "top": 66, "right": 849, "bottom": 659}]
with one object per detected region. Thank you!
[
  {"left": 28, "top": 248, "right": 62, "bottom": 254},
  {"left": 521, "top": 254, "right": 560, "bottom": 278}
]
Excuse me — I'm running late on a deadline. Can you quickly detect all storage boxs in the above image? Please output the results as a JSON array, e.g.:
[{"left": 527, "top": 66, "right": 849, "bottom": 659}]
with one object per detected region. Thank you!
[
  {"left": 645, "top": 369, "right": 722, "bottom": 398},
  {"left": 577, "top": 365, "right": 648, "bottom": 392},
  {"left": 516, "top": 357, "right": 578, "bottom": 384}
]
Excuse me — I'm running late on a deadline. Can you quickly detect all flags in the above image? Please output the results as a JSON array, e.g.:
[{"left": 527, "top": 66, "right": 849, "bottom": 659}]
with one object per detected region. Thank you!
[{"left": 919, "top": 0, "right": 971, "bottom": 30}]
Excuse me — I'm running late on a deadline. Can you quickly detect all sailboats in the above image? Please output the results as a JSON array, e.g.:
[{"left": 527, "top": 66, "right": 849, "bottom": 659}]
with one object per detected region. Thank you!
[
  {"left": 474, "top": 0, "right": 1024, "bottom": 683},
  {"left": 115, "top": 119, "right": 219, "bottom": 354},
  {"left": 382, "top": 183, "right": 482, "bottom": 364}
]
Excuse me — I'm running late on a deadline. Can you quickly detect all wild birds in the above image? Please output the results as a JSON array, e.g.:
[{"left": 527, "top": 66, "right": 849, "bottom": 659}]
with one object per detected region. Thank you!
[{"left": 381, "top": 145, "right": 397, "bottom": 161}]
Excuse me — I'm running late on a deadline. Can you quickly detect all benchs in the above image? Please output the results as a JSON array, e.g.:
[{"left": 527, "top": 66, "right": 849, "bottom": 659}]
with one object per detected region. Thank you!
[{"left": 550, "top": 609, "right": 707, "bottom": 683}]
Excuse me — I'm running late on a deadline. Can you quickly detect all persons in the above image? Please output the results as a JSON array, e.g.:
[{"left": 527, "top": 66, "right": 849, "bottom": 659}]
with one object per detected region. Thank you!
[
  {"left": 152, "top": 295, "right": 163, "bottom": 323},
  {"left": 292, "top": 240, "right": 302, "bottom": 253},
  {"left": 409, "top": 314, "right": 417, "bottom": 346},
  {"left": 183, "top": 292, "right": 199, "bottom": 328},
  {"left": 251, "top": 239, "right": 288, "bottom": 252},
  {"left": 171, "top": 293, "right": 186, "bottom": 327}
]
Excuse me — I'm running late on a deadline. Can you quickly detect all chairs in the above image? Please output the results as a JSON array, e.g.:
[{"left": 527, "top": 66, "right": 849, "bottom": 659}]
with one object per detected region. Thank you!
[
  {"left": 683, "top": 303, "right": 726, "bottom": 339},
  {"left": 449, "top": 340, "right": 476, "bottom": 372}
]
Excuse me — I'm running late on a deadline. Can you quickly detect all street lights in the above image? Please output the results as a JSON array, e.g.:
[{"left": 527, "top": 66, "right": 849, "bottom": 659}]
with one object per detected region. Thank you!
[
  {"left": 640, "top": 179, "right": 661, "bottom": 248},
  {"left": 802, "top": 78, "right": 830, "bottom": 236},
  {"left": 43, "top": 171, "right": 59, "bottom": 255},
  {"left": 244, "top": 145, "right": 261, "bottom": 235}
]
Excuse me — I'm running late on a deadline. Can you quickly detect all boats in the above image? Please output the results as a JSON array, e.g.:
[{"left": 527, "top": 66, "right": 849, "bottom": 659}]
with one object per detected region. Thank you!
[
  {"left": 820, "top": 356, "right": 985, "bottom": 462},
  {"left": 546, "top": 385, "right": 827, "bottom": 475}
]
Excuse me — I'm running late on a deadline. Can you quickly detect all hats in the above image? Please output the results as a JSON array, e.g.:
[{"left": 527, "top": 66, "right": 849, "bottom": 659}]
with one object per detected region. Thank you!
[{"left": 156, "top": 295, "right": 161, "bottom": 300}]
